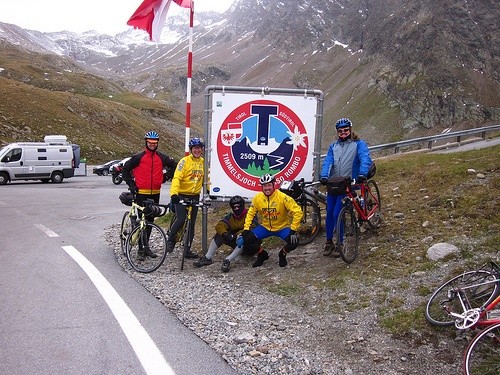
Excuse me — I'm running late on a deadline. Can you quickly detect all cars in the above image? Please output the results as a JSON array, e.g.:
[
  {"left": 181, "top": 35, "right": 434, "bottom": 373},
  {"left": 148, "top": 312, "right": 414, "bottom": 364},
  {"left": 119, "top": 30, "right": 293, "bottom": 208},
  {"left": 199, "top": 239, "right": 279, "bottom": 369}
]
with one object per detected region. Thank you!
[
  {"left": 108, "top": 157, "right": 134, "bottom": 173},
  {"left": 92, "top": 160, "right": 120, "bottom": 176}
]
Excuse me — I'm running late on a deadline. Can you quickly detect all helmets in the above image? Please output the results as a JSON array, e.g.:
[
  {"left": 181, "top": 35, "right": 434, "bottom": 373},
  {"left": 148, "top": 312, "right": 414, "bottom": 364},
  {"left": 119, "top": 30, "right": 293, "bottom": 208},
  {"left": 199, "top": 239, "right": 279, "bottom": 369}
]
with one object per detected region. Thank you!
[
  {"left": 189, "top": 137, "right": 204, "bottom": 148},
  {"left": 144, "top": 130, "right": 159, "bottom": 143},
  {"left": 259, "top": 173, "right": 275, "bottom": 185},
  {"left": 335, "top": 117, "right": 353, "bottom": 130},
  {"left": 229, "top": 195, "right": 245, "bottom": 208}
]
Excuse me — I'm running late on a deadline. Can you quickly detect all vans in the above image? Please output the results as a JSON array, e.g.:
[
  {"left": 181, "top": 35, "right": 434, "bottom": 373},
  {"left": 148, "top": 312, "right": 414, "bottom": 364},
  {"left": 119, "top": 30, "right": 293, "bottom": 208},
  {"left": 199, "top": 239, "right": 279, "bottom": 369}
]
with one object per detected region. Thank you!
[{"left": 0, "top": 135, "right": 74, "bottom": 186}]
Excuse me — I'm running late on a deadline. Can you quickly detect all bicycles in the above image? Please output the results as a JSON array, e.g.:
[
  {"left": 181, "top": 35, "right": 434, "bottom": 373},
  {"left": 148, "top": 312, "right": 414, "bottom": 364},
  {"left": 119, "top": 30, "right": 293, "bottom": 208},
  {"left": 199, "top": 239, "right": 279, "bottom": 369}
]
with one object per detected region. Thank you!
[
  {"left": 425, "top": 257, "right": 500, "bottom": 375},
  {"left": 166, "top": 196, "right": 211, "bottom": 270},
  {"left": 118, "top": 186, "right": 170, "bottom": 274},
  {"left": 279, "top": 177, "right": 369, "bottom": 246},
  {"left": 319, "top": 174, "right": 382, "bottom": 264}
]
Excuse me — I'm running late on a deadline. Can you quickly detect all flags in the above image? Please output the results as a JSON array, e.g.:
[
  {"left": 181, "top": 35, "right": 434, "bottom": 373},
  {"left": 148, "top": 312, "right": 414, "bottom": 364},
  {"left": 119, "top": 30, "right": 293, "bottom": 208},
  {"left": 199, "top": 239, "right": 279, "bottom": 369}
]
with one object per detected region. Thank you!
[{"left": 127, "top": 0, "right": 194, "bottom": 48}]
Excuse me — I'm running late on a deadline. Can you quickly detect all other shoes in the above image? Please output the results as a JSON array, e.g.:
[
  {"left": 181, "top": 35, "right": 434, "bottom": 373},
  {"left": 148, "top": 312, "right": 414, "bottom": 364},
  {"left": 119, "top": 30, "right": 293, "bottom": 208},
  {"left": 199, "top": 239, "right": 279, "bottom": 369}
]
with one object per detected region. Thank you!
[
  {"left": 252, "top": 249, "right": 269, "bottom": 267},
  {"left": 221, "top": 258, "right": 232, "bottom": 273},
  {"left": 137, "top": 247, "right": 157, "bottom": 260},
  {"left": 278, "top": 248, "right": 287, "bottom": 267},
  {"left": 193, "top": 255, "right": 212, "bottom": 267},
  {"left": 332, "top": 242, "right": 345, "bottom": 258},
  {"left": 166, "top": 241, "right": 174, "bottom": 252},
  {"left": 184, "top": 250, "right": 198, "bottom": 258},
  {"left": 322, "top": 241, "right": 335, "bottom": 256}
]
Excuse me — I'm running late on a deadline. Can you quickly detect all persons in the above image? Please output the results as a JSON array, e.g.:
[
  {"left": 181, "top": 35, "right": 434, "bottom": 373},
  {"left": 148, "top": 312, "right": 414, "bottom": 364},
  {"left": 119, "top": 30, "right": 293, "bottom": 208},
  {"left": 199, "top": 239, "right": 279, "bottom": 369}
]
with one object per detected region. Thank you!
[
  {"left": 320, "top": 118, "right": 372, "bottom": 256},
  {"left": 193, "top": 195, "right": 259, "bottom": 272},
  {"left": 123, "top": 130, "right": 178, "bottom": 261},
  {"left": 237, "top": 174, "right": 304, "bottom": 268},
  {"left": 166, "top": 137, "right": 217, "bottom": 259}
]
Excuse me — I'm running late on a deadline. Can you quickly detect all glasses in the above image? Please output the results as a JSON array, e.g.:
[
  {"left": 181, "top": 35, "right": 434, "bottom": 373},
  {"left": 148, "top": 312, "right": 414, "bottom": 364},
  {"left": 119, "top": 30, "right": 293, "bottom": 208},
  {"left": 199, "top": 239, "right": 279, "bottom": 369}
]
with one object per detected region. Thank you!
[{"left": 338, "top": 128, "right": 350, "bottom": 133}]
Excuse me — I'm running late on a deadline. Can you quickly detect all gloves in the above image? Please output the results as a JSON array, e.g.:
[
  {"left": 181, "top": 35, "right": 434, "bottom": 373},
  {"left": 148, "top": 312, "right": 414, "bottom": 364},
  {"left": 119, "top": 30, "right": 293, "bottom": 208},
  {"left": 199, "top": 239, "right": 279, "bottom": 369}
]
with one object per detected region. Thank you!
[
  {"left": 356, "top": 175, "right": 365, "bottom": 184},
  {"left": 162, "top": 173, "right": 170, "bottom": 183},
  {"left": 223, "top": 231, "right": 233, "bottom": 242},
  {"left": 290, "top": 234, "right": 298, "bottom": 245},
  {"left": 130, "top": 186, "right": 138, "bottom": 195},
  {"left": 321, "top": 178, "right": 328, "bottom": 186},
  {"left": 242, "top": 230, "right": 249, "bottom": 238},
  {"left": 171, "top": 195, "right": 180, "bottom": 205}
]
